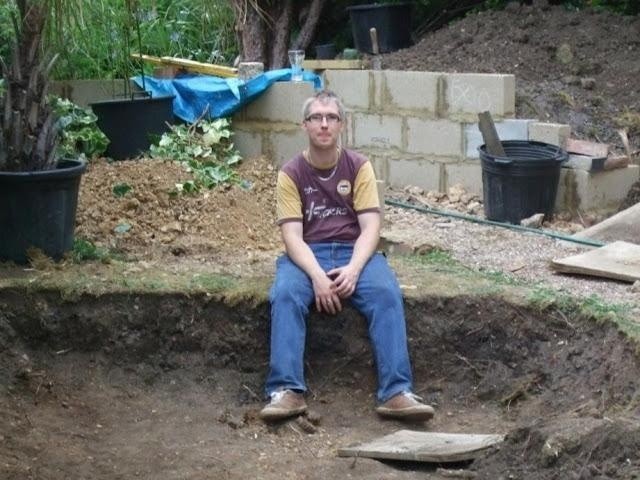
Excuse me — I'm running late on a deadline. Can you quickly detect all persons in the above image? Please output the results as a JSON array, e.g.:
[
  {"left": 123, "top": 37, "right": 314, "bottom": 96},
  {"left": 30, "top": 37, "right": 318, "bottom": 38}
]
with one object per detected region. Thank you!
[{"left": 260, "top": 91, "right": 435, "bottom": 421}]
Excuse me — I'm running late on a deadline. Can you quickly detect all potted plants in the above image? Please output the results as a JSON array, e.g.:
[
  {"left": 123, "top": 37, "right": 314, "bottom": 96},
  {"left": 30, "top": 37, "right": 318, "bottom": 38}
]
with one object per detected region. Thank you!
[
  {"left": 1, "top": 0, "right": 87, "bottom": 270},
  {"left": 86, "top": 1, "right": 177, "bottom": 161}
]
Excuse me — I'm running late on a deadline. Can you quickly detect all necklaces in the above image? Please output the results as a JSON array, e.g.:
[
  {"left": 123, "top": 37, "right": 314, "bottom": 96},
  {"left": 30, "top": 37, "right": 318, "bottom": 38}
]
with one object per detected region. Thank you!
[{"left": 308, "top": 148, "right": 340, "bottom": 182}]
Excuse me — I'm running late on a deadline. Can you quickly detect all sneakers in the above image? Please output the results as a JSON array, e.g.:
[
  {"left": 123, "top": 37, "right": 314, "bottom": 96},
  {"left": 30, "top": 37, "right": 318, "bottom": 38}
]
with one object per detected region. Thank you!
[
  {"left": 376, "top": 391, "right": 434, "bottom": 415},
  {"left": 260, "top": 389, "right": 307, "bottom": 419}
]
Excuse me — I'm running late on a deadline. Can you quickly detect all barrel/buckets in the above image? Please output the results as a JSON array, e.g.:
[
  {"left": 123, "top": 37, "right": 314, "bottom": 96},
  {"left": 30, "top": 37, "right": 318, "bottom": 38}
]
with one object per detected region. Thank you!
[
  {"left": 0, "top": 157, "right": 84, "bottom": 265},
  {"left": 89, "top": 87, "right": 178, "bottom": 159},
  {"left": 476, "top": 140, "right": 569, "bottom": 229}
]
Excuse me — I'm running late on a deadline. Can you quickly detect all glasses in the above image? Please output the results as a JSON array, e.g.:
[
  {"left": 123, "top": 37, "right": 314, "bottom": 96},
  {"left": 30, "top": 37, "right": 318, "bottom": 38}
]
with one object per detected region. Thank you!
[{"left": 305, "top": 113, "right": 341, "bottom": 122}]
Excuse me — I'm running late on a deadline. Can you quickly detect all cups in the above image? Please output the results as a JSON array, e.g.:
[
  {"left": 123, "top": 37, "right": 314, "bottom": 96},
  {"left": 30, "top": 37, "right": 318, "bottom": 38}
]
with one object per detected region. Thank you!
[{"left": 286, "top": 47, "right": 306, "bottom": 82}]
